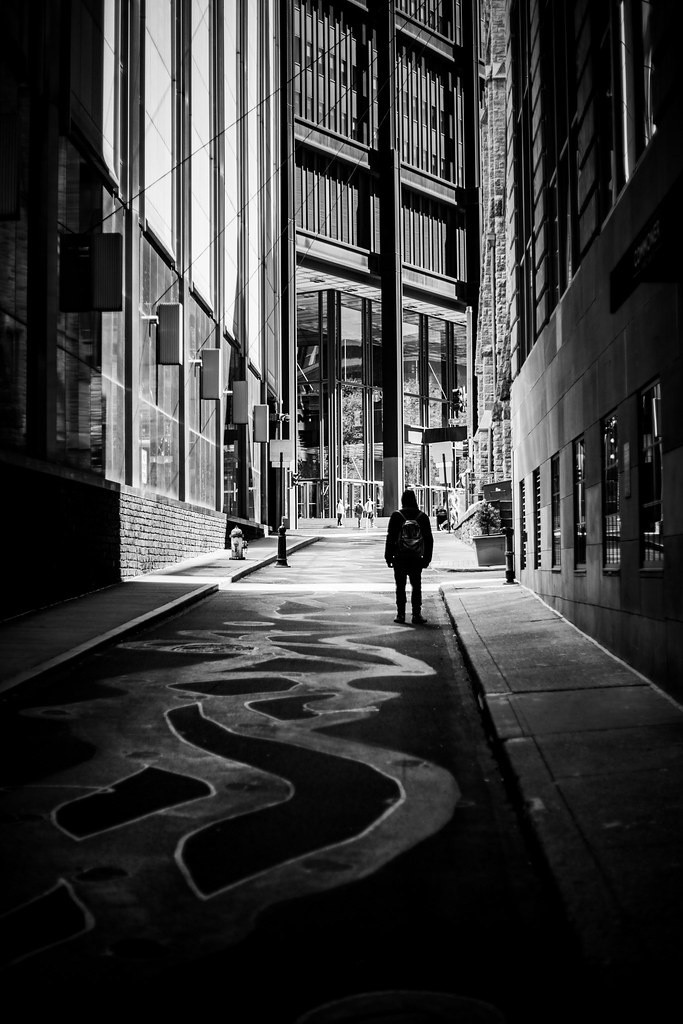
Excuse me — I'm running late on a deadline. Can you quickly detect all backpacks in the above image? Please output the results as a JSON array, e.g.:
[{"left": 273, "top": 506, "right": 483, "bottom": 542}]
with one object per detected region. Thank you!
[{"left": 393, "top": 510, "right": 425, "bottom": 558}]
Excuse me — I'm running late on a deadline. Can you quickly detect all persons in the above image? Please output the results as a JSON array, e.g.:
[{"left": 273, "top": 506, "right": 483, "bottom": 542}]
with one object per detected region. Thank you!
[
  {"left": 384, "top": 490, "right": 434, "bottom": 624},
  {"left": 354, "top": 496, "right": 377, "bottom": 528},
  {"left": 336, "top": 499, "right": 344, "bottom": 528}
]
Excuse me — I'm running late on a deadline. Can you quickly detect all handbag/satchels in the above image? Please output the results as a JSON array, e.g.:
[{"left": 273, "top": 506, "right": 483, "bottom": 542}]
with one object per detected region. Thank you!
[{"left": 366, "top": 512, "right": 373, "bottom": 519}]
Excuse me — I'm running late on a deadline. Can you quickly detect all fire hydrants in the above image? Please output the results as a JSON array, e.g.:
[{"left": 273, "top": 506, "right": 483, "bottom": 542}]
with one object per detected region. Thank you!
[{"left": 228, "top": 526, "right": 248, "bottom": 560}]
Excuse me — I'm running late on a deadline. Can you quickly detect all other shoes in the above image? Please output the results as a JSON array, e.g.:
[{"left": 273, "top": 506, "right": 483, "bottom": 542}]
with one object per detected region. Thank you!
[
  {"left": 439, "top": 525, "right": 443, "bottom": 530},
  {"left": 412, "top": 615, "right": 427, "bottom": 623},
  {"left": 394, "top": 616, "right": 405, "bottom": 623}
]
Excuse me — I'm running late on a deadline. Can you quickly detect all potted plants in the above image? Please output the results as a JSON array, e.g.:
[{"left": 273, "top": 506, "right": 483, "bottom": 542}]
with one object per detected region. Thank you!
[{"left": 472, "top": 502, "right": 507, "bottom": 567}]
